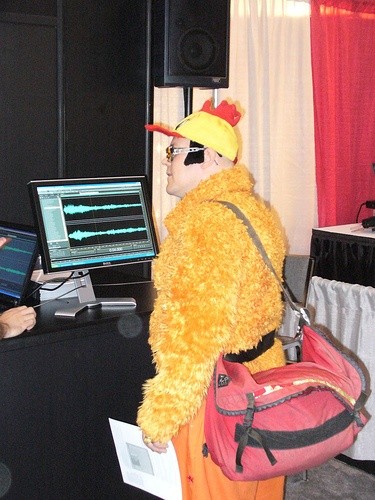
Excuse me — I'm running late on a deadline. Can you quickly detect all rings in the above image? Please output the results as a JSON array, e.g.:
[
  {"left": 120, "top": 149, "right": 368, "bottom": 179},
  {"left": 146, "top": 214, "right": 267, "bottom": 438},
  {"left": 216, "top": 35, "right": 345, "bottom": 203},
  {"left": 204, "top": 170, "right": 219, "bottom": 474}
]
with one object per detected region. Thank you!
[{"left": 143, "top": 437, "right": 151, "bottom": 444}]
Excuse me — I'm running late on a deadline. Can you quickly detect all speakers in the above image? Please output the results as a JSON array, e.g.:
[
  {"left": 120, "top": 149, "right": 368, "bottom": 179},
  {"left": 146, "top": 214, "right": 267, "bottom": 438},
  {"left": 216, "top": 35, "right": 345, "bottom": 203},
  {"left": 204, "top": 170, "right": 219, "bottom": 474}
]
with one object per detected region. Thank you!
[{"left": 151, "top": 0, "right": 230, "bottom": 90}]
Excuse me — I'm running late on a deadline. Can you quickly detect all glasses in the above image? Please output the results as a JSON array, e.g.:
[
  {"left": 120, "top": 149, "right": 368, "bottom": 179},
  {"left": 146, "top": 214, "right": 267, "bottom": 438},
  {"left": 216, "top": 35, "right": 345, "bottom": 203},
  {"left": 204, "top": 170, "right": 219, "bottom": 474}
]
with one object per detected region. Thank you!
[{"left": 165, "top": 144, "right": 207, "bottom": 163}]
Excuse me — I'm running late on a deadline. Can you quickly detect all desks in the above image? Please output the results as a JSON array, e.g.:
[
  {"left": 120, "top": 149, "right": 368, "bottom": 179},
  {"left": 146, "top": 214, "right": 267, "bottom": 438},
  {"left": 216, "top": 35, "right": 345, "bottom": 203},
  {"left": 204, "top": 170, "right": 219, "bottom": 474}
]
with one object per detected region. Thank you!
[{"left": 0, "top": 271, "right": 157, "bottom": 500}]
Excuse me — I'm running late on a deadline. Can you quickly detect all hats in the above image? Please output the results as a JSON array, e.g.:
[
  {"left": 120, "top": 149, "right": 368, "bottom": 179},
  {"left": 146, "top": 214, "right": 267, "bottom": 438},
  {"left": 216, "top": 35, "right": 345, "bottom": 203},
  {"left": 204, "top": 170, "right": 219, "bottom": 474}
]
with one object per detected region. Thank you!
[{"left": 144, "top": 98, "right": 242, "bottom": 163}]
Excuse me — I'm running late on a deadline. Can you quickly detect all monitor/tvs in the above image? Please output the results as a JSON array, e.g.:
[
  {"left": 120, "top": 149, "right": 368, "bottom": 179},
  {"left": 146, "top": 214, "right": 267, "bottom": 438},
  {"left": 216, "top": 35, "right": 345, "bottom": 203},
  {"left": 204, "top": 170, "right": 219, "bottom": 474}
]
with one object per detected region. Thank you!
[{"left": 28, "top": 174, "right": 159, "bottom": 317}]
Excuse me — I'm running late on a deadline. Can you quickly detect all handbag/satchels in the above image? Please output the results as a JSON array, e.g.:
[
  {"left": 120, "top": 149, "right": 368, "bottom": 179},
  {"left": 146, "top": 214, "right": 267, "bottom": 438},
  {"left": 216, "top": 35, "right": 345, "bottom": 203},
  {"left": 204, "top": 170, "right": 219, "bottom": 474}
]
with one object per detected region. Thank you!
[{"left": 202, "top": 324, "right": 372, "bottom": 483}]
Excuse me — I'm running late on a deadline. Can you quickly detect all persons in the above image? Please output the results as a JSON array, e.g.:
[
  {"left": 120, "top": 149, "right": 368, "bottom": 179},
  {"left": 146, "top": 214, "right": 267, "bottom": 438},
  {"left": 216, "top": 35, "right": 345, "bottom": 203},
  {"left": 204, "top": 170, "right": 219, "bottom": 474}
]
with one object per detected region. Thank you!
[
  {"left": 0, "top": 237, "right": 36, "bottom": 339},
  {"left": 137, "top": 100, "right": 290, "bottom": 500}
]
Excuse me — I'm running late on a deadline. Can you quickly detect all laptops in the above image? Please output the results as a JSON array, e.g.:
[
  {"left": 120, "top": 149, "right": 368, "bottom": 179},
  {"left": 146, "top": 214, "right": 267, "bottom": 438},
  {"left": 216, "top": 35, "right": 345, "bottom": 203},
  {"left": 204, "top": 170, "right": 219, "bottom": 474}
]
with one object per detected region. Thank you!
[{"left": 0, "top": 221, "right": 40, "bottom": 316}]
